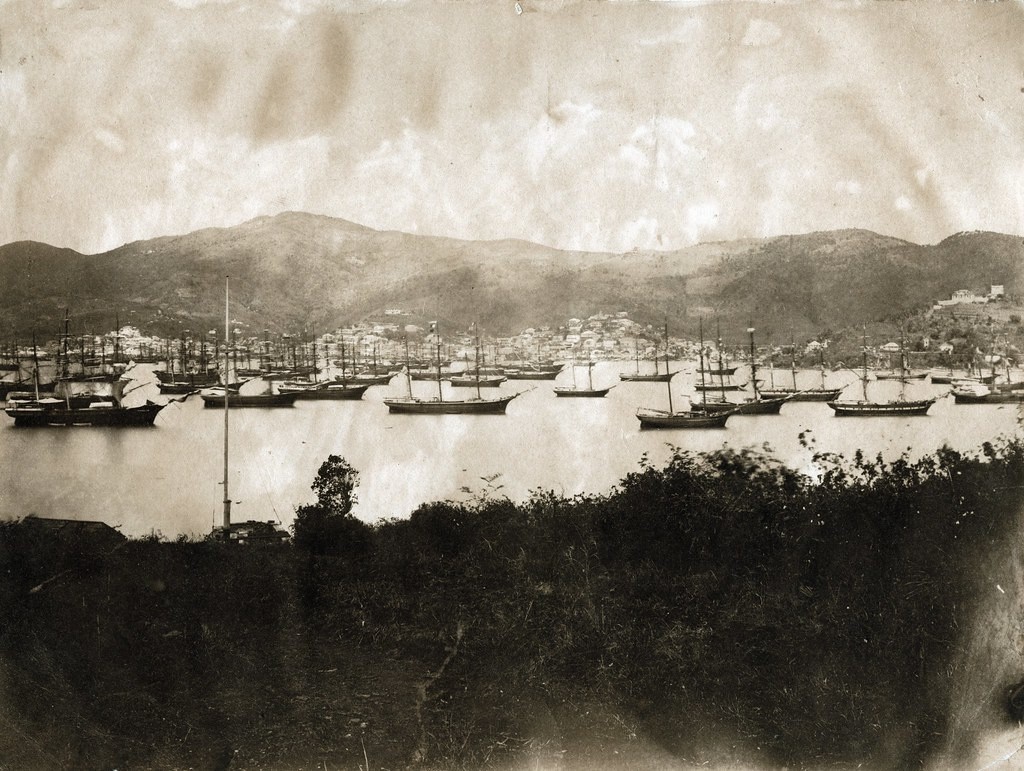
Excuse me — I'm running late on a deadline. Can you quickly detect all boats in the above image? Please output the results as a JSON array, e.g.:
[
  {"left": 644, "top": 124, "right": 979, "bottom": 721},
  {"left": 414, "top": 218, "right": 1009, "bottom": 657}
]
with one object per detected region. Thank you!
[
  {"left": 448, "top": 376, "right": 507, "bottom": 387},
  {"left": 875, "top": 373, "right": 929, "bottom": 380},
  {"left": 481, "top": 344, "right": 565, "bottom": 379},
  {"left": 950, "top": 381, "right": 1024, "bottom": 404},
  {"left": 404, "top": 371, "right": 466, "bottom": 382},
  {"left": 930, "top": 373, "right": 1001, "bottom": 384},
  {"left": 570, "top": 360, "right": 597, "bottom": 366}
]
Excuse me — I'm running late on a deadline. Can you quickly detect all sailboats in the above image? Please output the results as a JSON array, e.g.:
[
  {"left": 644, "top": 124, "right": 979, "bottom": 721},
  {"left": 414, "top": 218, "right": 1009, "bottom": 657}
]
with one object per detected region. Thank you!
[
  {"left": 695, "top": 318, "right": 737, "bottom": 375},
  {"left": 694, "top": 347, "right": 748, "bottom": 392},
  {"left": 332, "top": 336, "right": 451, "bottom": 386},
  {"left": 619, "top": 341, "right": 679, "bottom": 383},
  {"left": 689, "top": 318, "right": 802, "bottom": 416},
  {"left": 0, "top": 309, "right": 201, "bottom": 428},
  {"left": 553, "top": 347, "right": 619, "bottom": 398},
  {"left": 635, "top": 316, "right": 742, "bottom": 429},
  {"left": 153, "top": 327, "right": 370, "bottom": 409},
  {"left": 382, "top": 321, "right": 521, "bottom": 414},
  {"left": 827, "top": 320, "right": 936, "bottom": 416},
  {"left": 757, "top": 336, "right": 844, "bottom": 402}
]
